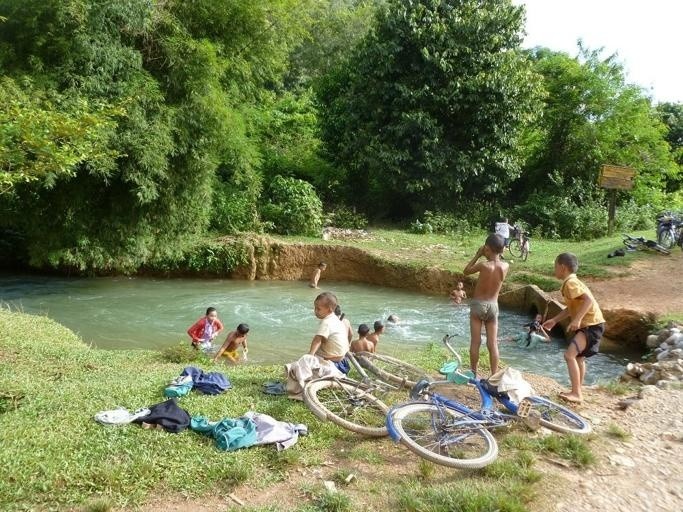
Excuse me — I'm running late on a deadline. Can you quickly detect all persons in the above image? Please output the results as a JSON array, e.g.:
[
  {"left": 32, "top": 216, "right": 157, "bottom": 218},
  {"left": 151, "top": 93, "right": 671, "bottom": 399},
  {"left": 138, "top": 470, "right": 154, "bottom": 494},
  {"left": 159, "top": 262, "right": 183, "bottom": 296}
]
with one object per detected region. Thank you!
[
  {"left": 308, "top": 262, "right": 327, "bottom": 289},
  {"left": 463, "top": 234, "right": 509, "bottom": 377},
  {"left": 307, "top": 292, "right": 400, "bottom": 373},
  {"left": 493, "top": 217, "right": 512, "bottom": 259},
  {"left": 187, "top": 307, "right": 250, "bottom": 367},
  {"left": 506, "top": 300, "right": 552, "bottom": 352},
  {"left": 540, "top": 253, "right": 608, "bottom": 403},
  {"left": 449, "top": 281, "right": 467, "bottom": 304}
]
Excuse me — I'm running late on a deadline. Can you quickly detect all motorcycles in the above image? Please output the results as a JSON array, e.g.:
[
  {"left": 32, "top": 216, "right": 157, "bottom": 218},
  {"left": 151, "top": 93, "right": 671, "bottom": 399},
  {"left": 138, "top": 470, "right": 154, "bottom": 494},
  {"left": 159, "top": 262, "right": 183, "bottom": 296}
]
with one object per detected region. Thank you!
[{"left": 655, "top": 211, "right": 683, "bottom": 249}]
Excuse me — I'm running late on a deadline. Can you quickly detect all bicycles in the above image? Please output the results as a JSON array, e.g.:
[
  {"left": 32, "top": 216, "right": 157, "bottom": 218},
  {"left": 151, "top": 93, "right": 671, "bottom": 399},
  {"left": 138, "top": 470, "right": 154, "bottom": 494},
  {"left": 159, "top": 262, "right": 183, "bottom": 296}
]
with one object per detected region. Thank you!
[
  {"left": 289, "top": 311, "right": 436, "bottom": 437},
  {"left": 385, "top": 332, "right": 594, "bottom": 470},
  {"left": 509, "top": 223, "right": 532, "bottom": 262},
  {"left": 623, "top": 233, "right": 671, "bottom": 256}
]
